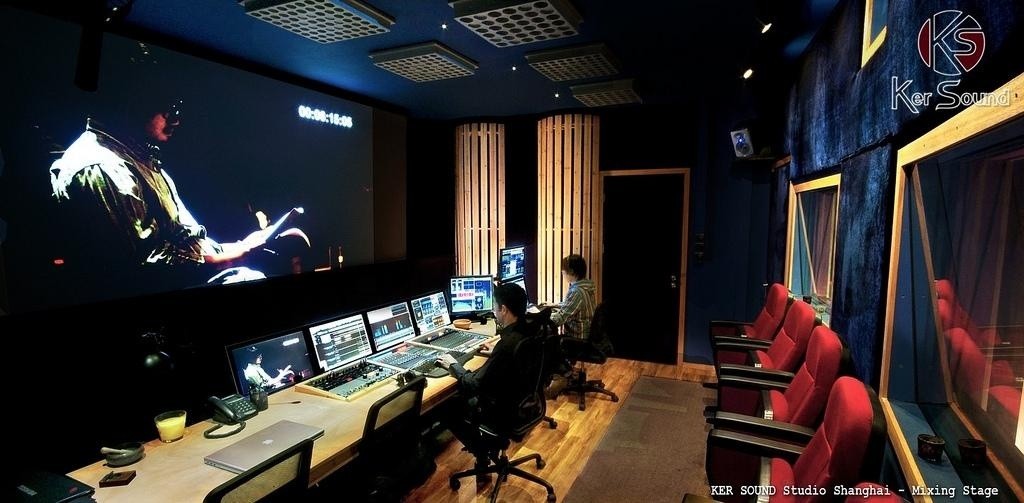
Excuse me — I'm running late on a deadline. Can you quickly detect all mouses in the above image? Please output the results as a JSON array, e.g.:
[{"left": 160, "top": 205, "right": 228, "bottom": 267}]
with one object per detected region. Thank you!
[{"left": 477, "top": 346, "right": 488, "bottom": 353}]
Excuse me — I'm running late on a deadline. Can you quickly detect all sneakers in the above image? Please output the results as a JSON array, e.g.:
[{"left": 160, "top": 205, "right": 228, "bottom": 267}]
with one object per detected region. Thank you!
[
  {"left": 544, "top": 376, "right": 568, "bottom": 399},
  {"left": 572, "top": 367, "right": 581, "bottom": 380}
]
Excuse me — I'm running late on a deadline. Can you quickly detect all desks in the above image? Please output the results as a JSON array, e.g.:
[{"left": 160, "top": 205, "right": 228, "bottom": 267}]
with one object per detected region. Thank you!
[{"left": 68, "top": 314, "right": 498, "bottom": 502}]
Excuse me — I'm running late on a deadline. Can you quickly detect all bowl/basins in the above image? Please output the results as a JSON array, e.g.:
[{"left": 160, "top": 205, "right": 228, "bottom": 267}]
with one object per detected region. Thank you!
[{"left": 106, "top": 443, "right": 146, "bottom": 467}]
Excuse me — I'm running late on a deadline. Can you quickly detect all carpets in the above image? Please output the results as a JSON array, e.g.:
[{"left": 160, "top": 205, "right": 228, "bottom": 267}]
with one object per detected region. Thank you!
[{"left": 561, "top": 371, "right": 718, "bottom": 502}]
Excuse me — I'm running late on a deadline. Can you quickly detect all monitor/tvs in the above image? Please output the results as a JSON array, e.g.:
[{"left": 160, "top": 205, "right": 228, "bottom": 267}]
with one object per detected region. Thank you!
[
  {"left": 447, "top": 275, "right": 492, "bottom": 320},
  {"left": 223, "top": 328, "right": 317, "bottom": 396},
  {"left": 304, "top": 310, "right": 372, "bottom": 372},
  {"left": 498, "top": 244, "right": 525, "bottom": 285},
  {"left": 364, "top": 299, "right": 418, "bottom": 352},
  {"left": 407, "top": 287, "right": 452, "bottom": 338}
]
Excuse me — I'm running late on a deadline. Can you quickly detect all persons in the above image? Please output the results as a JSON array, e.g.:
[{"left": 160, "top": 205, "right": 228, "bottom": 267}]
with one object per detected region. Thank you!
[
  {"left": 43, "top": 59, "right": 313, "bottom": 293},
  {"left": 230, "top": 346, "right": 296, "bottom": 393},
  {"left": 545, "top": 253, "right": 598, "bottom": 398},
  {"left": 433, "top": 280, "right": 544, "bottom": 484}
]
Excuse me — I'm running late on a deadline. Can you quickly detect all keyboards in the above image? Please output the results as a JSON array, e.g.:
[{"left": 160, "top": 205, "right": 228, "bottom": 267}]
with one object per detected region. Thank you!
[
  {"left": 412, "top": 350, "right": 473, "bottom": 377},
  {"left": 528, "top": 303, "right": 557, "bottom": 319}
]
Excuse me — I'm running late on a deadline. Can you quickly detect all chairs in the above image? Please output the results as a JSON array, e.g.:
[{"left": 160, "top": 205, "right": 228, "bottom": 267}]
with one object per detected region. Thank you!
[{"left": 934, "top": 276, "right": 1018, "bottom": 449}]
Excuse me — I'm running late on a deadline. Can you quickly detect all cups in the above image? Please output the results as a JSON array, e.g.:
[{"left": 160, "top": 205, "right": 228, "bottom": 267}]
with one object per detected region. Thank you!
[{"left": 154, "top": 409, "right": 188, "bottom": 443}]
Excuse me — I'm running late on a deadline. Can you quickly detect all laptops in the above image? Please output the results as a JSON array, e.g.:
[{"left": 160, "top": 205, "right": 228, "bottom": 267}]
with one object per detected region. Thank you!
[{"left": 203, "top": 419, "right": 324, "bottom": 473}]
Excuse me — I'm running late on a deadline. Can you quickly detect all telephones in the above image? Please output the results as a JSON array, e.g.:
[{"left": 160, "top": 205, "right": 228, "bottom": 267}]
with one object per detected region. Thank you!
[{"left": 209, "top": 392, "right": 257, "bottom": 425}]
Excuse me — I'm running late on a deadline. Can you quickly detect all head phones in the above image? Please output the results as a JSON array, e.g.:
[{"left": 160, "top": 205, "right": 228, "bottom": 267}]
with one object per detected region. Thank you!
[
  {"left": 396, "top": 371, "right": 428, "bottom": 388},
  {"left": 86, "top": 126, "right": 165, "bottom": 173}
]
